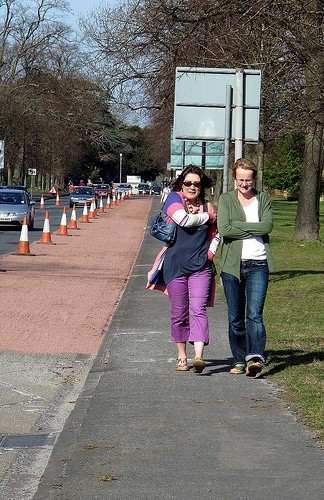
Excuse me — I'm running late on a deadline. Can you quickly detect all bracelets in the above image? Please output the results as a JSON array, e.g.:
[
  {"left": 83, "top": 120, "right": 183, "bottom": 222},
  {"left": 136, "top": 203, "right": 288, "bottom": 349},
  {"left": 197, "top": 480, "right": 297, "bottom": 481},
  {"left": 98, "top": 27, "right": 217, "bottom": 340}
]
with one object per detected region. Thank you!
[{"left": 211, "top": 211, "right": 217, "bottom": 224}]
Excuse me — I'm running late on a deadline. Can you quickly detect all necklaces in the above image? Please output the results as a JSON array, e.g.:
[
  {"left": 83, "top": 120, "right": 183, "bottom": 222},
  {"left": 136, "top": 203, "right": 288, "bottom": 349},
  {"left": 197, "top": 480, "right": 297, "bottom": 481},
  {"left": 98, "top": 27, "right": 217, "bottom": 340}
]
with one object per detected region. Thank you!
[{"left": 184, "top": 191, "right": 201, "bottom": 214}]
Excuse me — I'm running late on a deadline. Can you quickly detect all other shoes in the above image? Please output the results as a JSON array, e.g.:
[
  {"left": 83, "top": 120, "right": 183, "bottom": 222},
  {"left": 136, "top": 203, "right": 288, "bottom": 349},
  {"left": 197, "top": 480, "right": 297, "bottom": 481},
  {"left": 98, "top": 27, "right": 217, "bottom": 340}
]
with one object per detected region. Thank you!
[
  {"left": 176, "top": 357, "right": 188, "bottom": 370},
  {"left": 192, "top": 358, "right": 205, "bottom": 373},
  {"left": 228, "top": 366, "right": 244, "bottom": 374},
  {"left": 246, "top": 360, "right": 262, "bottom": 377}
]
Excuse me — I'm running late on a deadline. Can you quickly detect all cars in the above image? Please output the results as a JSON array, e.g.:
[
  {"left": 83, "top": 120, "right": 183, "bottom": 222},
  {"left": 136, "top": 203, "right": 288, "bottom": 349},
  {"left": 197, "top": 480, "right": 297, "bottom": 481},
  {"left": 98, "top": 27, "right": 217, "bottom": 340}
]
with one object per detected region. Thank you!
[
  {"left": 138, "top": 183, "right": 162, "bottom": 195},
  {"left": 0, "top": 185, "right": 34, "bottom": 231},
  {"left": 69, "top": 186, "right": 97, "bottom": 208},
  {"left": 95, "top": 184, "right": 112, "bottom": 197},
  {"left": 116, "top": 184, "right": 133, "bottom": 195}
]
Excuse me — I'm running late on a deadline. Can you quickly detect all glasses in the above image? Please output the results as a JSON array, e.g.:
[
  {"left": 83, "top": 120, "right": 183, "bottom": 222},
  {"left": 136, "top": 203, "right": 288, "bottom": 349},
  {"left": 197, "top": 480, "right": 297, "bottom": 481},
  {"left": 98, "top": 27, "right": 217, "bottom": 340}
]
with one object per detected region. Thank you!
[
  {"left": 183, "top": 181, "right": 200, "bottom": 187},
  {"left": 235, "top": 178, "right": 253, "bottom": 183}
]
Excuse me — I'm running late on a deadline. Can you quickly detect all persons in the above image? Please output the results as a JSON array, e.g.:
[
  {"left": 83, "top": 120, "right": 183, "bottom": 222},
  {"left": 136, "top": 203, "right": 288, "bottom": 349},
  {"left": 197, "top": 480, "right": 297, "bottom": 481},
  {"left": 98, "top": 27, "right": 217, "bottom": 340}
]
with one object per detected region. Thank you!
[
  {"left": 159, "top": 165, "right": 221, "bottom": 373},
  {"left": 68, "top": 178, "right": 93, "bottom": 192},
  {"left": 217, "top": 158, "right": 273, "bottom": 377}
]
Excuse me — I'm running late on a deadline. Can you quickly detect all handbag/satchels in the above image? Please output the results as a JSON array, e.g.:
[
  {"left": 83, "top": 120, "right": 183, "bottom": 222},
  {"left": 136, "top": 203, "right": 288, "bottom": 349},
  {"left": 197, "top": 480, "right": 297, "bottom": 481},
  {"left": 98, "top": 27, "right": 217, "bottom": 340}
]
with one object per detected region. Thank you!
[{"left": 150, "top": 192, "right": 182, "bottom": 244}]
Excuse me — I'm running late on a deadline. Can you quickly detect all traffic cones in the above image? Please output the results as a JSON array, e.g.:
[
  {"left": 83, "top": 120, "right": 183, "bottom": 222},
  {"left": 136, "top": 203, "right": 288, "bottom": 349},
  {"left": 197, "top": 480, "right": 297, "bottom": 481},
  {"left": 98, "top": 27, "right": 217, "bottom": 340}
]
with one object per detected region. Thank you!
[
  {"left": 36, "top": 210, "right": 56, "bottom": 245},
  {"left": 79, "top": 201, "right": 92, "bottom": 223},
  {"left": 98, "top": 195, "right": 107, "bottom": 213},
  {"left": 106, "top": 194, "right": 113, "bottom": 209},
  {"left": 66, "top": 204, "right": 81, "bottom": 229},
  {"left": 11, "top": 215, "right": 36, "bottom": 256},
  {"left": 113, "top": 190, "right": 128, "bottom": 205},
  {"left": 55, "top": 189, "right": 63, "bottom": 206},
  {"left": 40, "top": 193, "right": 47, "bottom": 210},
  {"left": 88, "top": 198, "right": 99, "bottom": 219},
  {"left": 55, "top": 206, "right": 72, "bottom": 236}
]
